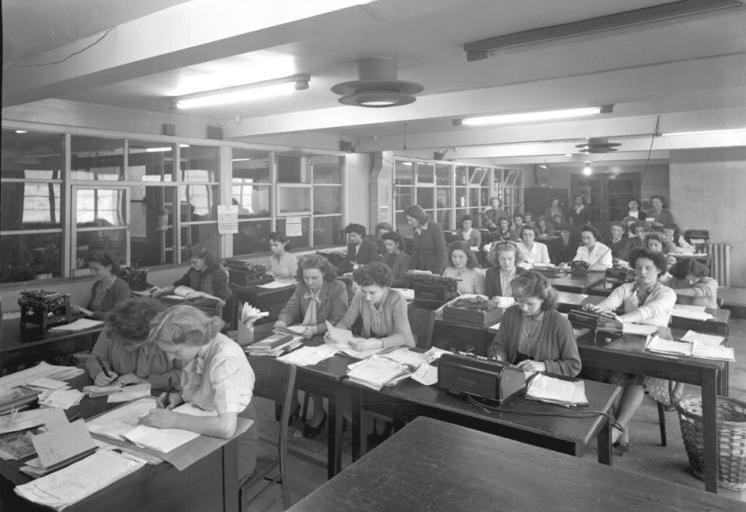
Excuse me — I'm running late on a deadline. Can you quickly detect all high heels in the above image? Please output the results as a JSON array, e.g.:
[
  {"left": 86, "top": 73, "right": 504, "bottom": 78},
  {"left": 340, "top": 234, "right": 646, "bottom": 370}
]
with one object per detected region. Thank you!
[
  {"left": 374, "top": 421, "right": 393, "bottom": 442},
  {"left": 614, "top": 441, "right": 631, "bottom": 457},
  {"left": 367, "top": 419, "right": 376, "bottom": 444},
  {"left": 288, "top": 403, "right": 301, "bottom": 425},
  {"left": 665, "top": 391, "right": 681, "bottom": 412},
  {"left": 303, "top": 410, "right": 327, "bottom": 438}
]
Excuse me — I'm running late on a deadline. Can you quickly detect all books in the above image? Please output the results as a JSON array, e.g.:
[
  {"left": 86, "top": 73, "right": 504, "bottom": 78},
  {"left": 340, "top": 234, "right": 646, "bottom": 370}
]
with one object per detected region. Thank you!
[
  {"left": 348, "top": 351, "right": 430, "bottom": 394},
  {"left": 19, "top": 418, "right": 98, "bottom": 478},
  {"left": 245, "top": 334, "right": 303, "bottom": 357},
  {"left": 84, "top": 398, "right": 219, "bottom": 454}
]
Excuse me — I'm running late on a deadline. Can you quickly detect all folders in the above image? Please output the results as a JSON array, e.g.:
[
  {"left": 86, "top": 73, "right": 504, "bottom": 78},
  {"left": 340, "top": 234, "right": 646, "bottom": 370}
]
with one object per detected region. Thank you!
[{"left": 83, "top": 394, "right": 254, "bottom": 471}]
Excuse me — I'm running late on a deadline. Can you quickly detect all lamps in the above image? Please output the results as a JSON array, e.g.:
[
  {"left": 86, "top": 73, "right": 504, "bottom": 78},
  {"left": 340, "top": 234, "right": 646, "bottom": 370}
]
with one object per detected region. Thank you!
[
  {"left": 580, "top": 163, "right": 592, "bottom": 177},
  {"left": 169, "top": 75, "right": 312, "bottom": 110},
  {"left": 450, "top": 105, "right": 615, "bottom": 129}
]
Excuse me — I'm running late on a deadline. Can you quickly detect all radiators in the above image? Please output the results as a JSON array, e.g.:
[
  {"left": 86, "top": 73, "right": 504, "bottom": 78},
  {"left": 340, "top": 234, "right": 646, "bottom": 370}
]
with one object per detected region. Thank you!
[{"left": 689, "top": 245, "right": 732, "bottom": 290}]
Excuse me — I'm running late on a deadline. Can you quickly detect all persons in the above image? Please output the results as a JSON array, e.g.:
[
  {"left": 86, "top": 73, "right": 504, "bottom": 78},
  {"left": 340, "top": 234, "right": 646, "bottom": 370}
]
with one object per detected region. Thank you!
[
  {"left": 662, "top": 223, "right": 693, "bottom": 254},
  {"left": 666, "top": 258, "right": 719, "bottom": 308},
  {"left": 440, "top": 241, "right": 485, "bottom": 295},
  {"left": 516, "top": 224, "right": 551, "bottom": 265},
  {"left": 581, "top": 248, "right": 677, "bottom": 455},
  {"left": 629, "top": 220, "right": 650, "bottom": 251},
  {"left": 323, "top": 262, "right": 416, "bottom": 351},
  {"left": 403, "top": 204, "right": 448, "bottom": 274},
  {"left": 623, "top": 216, "right": 637, "bottom": 225},
  {"left": 267, "top": 232, "right": 298, "bottom": 277},
  {"left": 173, "top": 245, "right": 231, "bottom": 305},
  {"left": 85, "top": 299, "right": 182, "bottom": 388},
  {"left": 484, "top": 197, "right": 507, "bottom": 232},
  {"left": 455, "top": 215, "right": 482, "bottom": 248},
  {"left": 489, "top": 270, "right": 583, "bottom": 372},
  {"left": 71, "top": 249, "right": 132, "bottom": 319},
  {"left": 645, "top": 196, "right": 672, "bottom": 232},
  {"left": 568, "top": 224, "right": 612, "bottom": 269},
  {"left": 273, "top": 253, "right": 349, "bottom": 439},
  {"left": 485, "top": 241, "right": 529, "bottom": 300},
  {"left": 570, "top": 193, "right": 590, "bottom": 228},
  {"left": 498, "top": 217, "right": 517, "bottom": 243},
  {"left": 137, "top": 304, "right": 260, "bottom": 484},
  {"left": 512, "top": 192, "right": 570, "bottom": 233},
  {"left": 344, "top": 224, "right": 377, "bottom": 266},
  {"left": 626, "top": 199, "right": 647, "bottom": 220},
  {"left": 643, "top": 232, "right": 677, "bottom": 279},
  {"left": 375, "top": 222, "right": 406, "bottom": 253},
  {"left": 381, "top": 232, "right": 412, "bottom": 279},
  {"left": 606, "top": 222, "right": 630, "bottom": 257}
]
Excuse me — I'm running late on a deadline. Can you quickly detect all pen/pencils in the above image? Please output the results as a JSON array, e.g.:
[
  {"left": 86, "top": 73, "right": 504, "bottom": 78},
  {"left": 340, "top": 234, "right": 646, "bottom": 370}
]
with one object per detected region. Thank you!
[
  {"left": 95, "top": 355, "right": 112, "bottom": 377},
  {"left": 164, "top": 376, "right": 172, "bottom": 408}
]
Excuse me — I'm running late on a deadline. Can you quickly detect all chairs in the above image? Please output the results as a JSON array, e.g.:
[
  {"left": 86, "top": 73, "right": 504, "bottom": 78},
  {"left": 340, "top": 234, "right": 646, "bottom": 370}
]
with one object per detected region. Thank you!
[{"left": 235, "top": 351, "right": 296, "bottom": 512}]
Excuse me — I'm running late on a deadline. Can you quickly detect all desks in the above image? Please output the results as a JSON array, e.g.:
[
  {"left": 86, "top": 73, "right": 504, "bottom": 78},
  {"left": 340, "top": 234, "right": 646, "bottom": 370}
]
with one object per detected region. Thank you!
[
  {"left": 289, "top": 415, "right": 744, "bottom": 512},
  {"left": 537, "top": 235, "right": 561, "bottom": 242},
  {"left": 576, "top": 326, "right": 730, "bottom": 495},
  {"left": 590, "top": 275, "right": 626, "bottom": 295},
  {"left": 345, "top": 343, "right": 622, "bottom": 467},
  {"left": 540, "top": 264, "right": 607, "bottom": 296},
  {"left": 0, "top": 305, "right": 107, "bottom": 358},
  {"left": 233, "top": 319, "right": 361, "bottom": 484},
  {"left": 239, "top": 276, "right": 298, "bottom": 308},
  {"left": 403, "top": 286, "right": 460, "bottom": 310},
  {"left": 671, "top": 303, "right": 731, "bottom": 394},
  {"left": 546, "top": 286, "right": 591, "bottom": 311},
  {"left": 436, "top": 303, "right": 504, "bottom": 351},
  {"left": 1, "top": 368, "right": 252, "bottom": 511}
]
